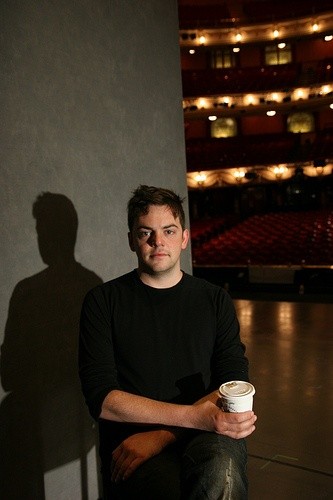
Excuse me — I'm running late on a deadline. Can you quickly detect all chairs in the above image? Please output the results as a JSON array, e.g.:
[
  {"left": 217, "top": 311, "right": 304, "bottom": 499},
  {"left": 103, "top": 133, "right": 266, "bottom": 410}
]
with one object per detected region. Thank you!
[{"left": 191, "top": 211, "right": 333, "bottom": 266}]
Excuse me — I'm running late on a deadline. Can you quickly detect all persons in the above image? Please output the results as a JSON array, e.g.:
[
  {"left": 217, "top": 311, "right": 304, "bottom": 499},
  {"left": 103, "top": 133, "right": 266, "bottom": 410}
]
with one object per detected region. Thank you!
[{"left": 79, "top": 184, "right": 258, "bottom": 500}]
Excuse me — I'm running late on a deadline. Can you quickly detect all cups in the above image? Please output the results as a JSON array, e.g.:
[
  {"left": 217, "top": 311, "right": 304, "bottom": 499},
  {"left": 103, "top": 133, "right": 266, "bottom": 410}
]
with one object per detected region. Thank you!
[{"left": 219, "top": 380, "right": 256, "bottom": 414}]
